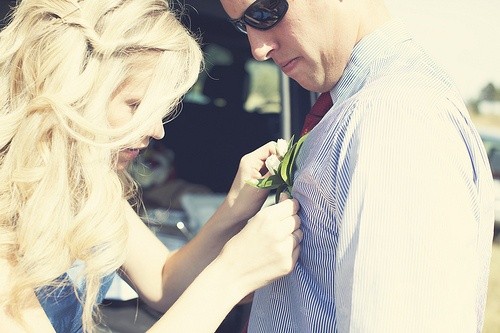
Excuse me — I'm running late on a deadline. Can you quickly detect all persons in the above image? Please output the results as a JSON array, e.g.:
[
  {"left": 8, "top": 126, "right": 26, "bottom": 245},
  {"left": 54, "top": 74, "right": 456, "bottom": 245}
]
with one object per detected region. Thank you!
[
  {"left": 219, "top": 0, "right": 496, "bottom": 333},
  {"left": 0, "top": 0, "right": 305, "bottom": 333}
]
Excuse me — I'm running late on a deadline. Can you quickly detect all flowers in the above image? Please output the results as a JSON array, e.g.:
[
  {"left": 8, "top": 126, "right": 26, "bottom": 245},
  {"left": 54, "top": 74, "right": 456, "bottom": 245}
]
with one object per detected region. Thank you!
[{"left": 247, "top": 134, "right": 309, "bottom": 202}]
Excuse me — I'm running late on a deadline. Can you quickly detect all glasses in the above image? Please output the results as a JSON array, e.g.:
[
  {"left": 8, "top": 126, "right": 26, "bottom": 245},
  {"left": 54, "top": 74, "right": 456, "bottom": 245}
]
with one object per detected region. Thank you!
[{"left": 228, "top": 0, "right": 290, "bottom": 36}]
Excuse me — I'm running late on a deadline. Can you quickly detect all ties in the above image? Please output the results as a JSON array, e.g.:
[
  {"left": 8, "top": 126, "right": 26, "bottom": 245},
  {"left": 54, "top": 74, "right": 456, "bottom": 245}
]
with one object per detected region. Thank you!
[{"left": 290, "top": 91, "right": 333, "bottom": 147}]
